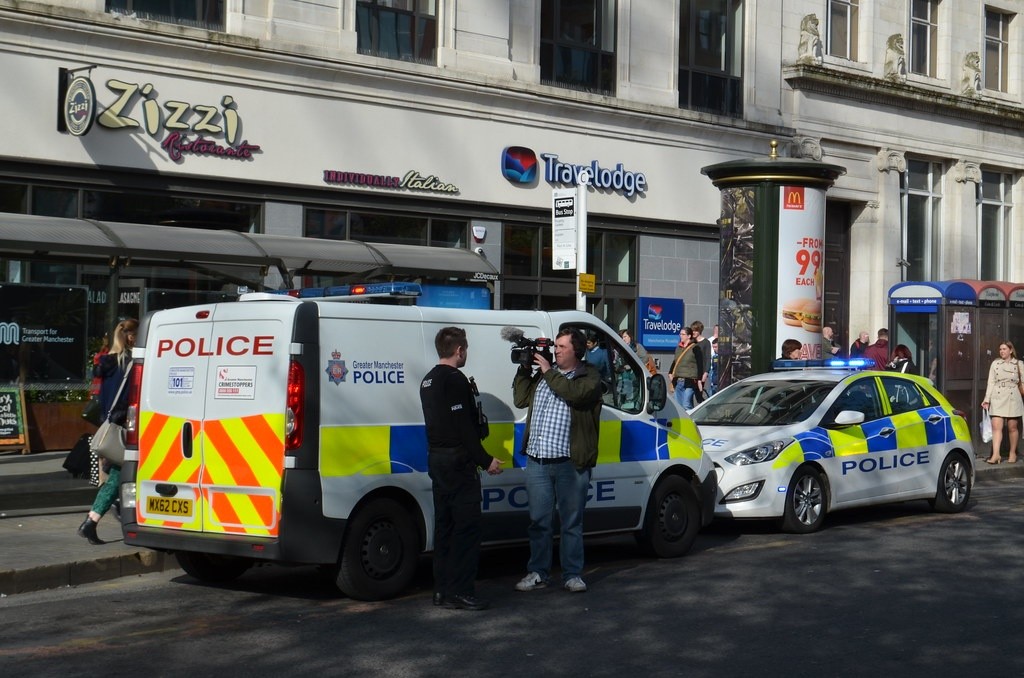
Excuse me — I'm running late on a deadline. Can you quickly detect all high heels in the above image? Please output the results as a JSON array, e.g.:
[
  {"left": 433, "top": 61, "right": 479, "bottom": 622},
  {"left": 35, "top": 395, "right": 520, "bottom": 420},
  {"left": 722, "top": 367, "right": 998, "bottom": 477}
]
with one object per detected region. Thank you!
[
  {"left": 987, "top": 456, "right": 1001, "bottom": 464},
  {"left": 1008, "top": 453, "right": 1017, "bottom": 463}
]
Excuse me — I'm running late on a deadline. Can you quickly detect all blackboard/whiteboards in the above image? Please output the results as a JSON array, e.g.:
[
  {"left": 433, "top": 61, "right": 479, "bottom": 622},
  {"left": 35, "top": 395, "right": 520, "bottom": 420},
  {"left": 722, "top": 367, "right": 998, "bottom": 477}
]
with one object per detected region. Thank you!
[
  {"left": 0, "top": 281, "right": 89, "bottom": 391},
  {"left": 0, "top": 383, "right": 30, "bottom": 450},
  {"left": 89, "top": 279, "right": 145, "bottom": 337}
]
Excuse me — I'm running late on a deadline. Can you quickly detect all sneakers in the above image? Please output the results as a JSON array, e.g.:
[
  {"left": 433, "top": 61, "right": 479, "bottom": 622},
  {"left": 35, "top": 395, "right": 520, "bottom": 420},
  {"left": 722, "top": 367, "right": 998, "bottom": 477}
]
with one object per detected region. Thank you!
[
  {"left": 564, "top": 577, "right": 586, "bottom": 593},
  {"left": 514, "top": 572, "right": 549, "bottom": 591}
]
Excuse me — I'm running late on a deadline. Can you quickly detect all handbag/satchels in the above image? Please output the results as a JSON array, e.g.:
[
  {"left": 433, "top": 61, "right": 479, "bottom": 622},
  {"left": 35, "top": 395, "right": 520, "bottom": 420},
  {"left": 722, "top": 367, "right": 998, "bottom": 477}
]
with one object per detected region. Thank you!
[
  {"left": 91, "top": 418, "right": 127, "bottom": 467},
  {"left": 644, "top": 355, "right": 657, "bottom": 376},
  {"left": 979, "top": 408, "right": 992, "bottom": 443},
  {"left": 63, "top": 433, "right": 110, "bottom": 488},
  {"left": 81, "top": 394, "right": 104, "bottom": 428},
  {"left": 669, "top": 372, "right": 675, "bottom": 393},
  {"left": 1017, "top": 382, "right": 1024, "bottom": 397}
]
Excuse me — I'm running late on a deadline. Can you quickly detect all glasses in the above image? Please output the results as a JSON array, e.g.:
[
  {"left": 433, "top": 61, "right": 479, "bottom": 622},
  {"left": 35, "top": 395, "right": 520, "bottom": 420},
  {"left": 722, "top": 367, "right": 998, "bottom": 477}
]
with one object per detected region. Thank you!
[{"left": 679, "top": 333, "right": 685, "bottom": 335}]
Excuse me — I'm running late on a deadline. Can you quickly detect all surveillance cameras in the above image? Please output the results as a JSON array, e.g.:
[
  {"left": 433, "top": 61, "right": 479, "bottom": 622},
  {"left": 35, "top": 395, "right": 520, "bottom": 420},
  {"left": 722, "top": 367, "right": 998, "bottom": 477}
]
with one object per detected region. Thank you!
[{"left": 474, "top": 247, "right": 485, "bottom": 255}]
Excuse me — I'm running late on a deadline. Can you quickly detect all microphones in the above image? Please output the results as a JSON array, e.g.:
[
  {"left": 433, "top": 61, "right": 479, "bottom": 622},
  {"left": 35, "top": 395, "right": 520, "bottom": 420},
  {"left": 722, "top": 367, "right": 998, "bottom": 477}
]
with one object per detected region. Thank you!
[{"left": 501, "top": 327, "right": 531, "bottom": 344}]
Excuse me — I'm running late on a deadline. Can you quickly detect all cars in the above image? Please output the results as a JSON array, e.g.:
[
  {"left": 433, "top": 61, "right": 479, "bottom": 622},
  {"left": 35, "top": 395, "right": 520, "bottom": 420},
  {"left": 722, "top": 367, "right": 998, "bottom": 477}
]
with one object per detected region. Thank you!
[{"left": 681, "top": 358, "right": 976, "bottom": 535}]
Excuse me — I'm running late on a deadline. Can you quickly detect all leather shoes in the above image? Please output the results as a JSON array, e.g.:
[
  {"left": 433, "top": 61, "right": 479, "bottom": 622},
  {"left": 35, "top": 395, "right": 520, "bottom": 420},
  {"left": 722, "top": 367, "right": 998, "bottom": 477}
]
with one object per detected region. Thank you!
[
  {"left": 78, "top": 514, "right": 105, "bottom": 545},
  {"left": 433, "top": 592, "right": 444, "bottom": 606},
  {"left": 444, "top": 591, "right": 489, "bottom": 610}
]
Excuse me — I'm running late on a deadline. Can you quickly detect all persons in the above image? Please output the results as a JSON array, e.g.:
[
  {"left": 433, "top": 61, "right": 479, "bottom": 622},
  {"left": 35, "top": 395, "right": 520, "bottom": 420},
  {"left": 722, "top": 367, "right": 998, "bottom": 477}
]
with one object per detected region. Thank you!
[
  {"left": 709, "top": 338, "right": 718, "bottom": 394},
  {"left": 981, "top": 341, "right": 1024, "bottom": 464},
  {"left": 887, "top": 345, "right": 919, "bottom": 375},
  {"left": 614, "top": 329, "right": 657, "bottom": 376},
  {"left": 514, "top": 327, "right": 604, "bottom": 591},
  {"left": 668, "top": 327, "right": 703, "bottom": 412},
  {"left": 822, "top": 327, "right": 841, "bottom": 358},
  {"left": 777, "top": 339, "right": 802, "bottom": 360},
  {"left": 709, "top": 325, "right": 718, "bottom": 356},
  {"left": 79, "top": 319, "right": 140, "bottom": 543},
  {"left": 864, "top": 328, "right": 888, "bottom": 370},
  {"left": 690, "top": 321, "right": 712, "bottom": 402},
  {"left": 420, "top": 327, "right": 506, "bottom": 610},
  {"left": 851, "top": 332, "right": 869, "bottom": 358},
  {"left": 90, "top": 336, "right": 110, "bottom": 400},
  {"left": 586, "top": 337, "right": 610, "bottom": 376}
]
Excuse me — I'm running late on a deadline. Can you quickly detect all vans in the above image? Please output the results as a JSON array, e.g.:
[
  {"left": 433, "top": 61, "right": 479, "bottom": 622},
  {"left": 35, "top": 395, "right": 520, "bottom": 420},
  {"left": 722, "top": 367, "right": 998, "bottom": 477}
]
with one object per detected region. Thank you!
[{"left": 119, "top": 283, "right": 718, "bottom": 601}]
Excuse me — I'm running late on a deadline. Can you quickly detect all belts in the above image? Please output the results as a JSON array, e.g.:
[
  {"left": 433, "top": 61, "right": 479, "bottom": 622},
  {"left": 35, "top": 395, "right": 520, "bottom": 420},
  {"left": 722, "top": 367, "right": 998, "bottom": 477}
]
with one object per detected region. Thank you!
[{"left": 528, "top": 455, "right": 570, "bottom": 465}]
